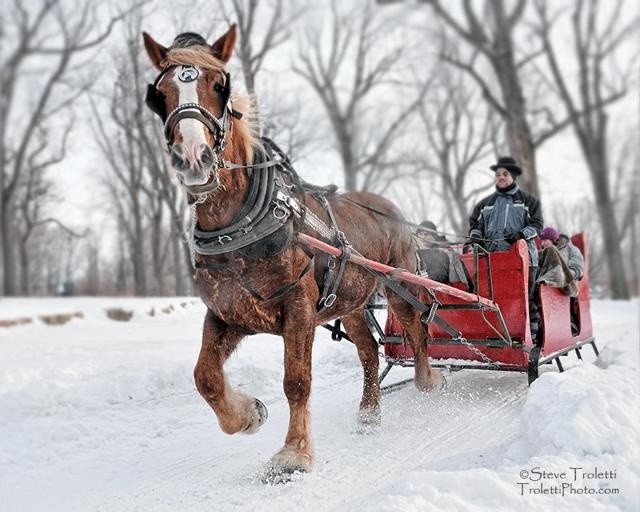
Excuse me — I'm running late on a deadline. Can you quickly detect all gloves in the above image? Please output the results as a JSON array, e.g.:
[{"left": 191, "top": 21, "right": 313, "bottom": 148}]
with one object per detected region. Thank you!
[
  {"left": 502, "top": 231, "right": 526, "bottom": 246},
  {"left": 466, "top": 233, "right": 486, "bottom": 248}
]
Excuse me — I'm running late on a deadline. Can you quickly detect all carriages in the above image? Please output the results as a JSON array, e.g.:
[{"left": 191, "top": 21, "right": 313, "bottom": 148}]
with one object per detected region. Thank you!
[{"left": 139, "top": 20, "right": 599, "bottom": 485}]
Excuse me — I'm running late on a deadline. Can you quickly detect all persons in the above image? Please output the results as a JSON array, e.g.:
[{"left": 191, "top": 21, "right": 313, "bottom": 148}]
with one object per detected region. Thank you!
[{"left": 415, "top": 157, "right": 584, "bottom": 348}]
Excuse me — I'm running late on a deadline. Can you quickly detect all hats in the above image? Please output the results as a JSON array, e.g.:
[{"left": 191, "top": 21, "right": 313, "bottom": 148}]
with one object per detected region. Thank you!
[
  {"left": 488, "top": 156, "right": 522, "bottom": 175},
  {"left": 543, "top": 226, "right": 558, "bottom": 241},
  {"left": 415, "top": 220, "right": 439, "bottom": 236}
]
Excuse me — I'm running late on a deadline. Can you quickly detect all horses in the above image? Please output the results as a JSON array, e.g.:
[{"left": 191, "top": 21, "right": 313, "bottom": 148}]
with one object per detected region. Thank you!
[{"left": 141, "top": 23, "right": 449, "bottom": 479}]
